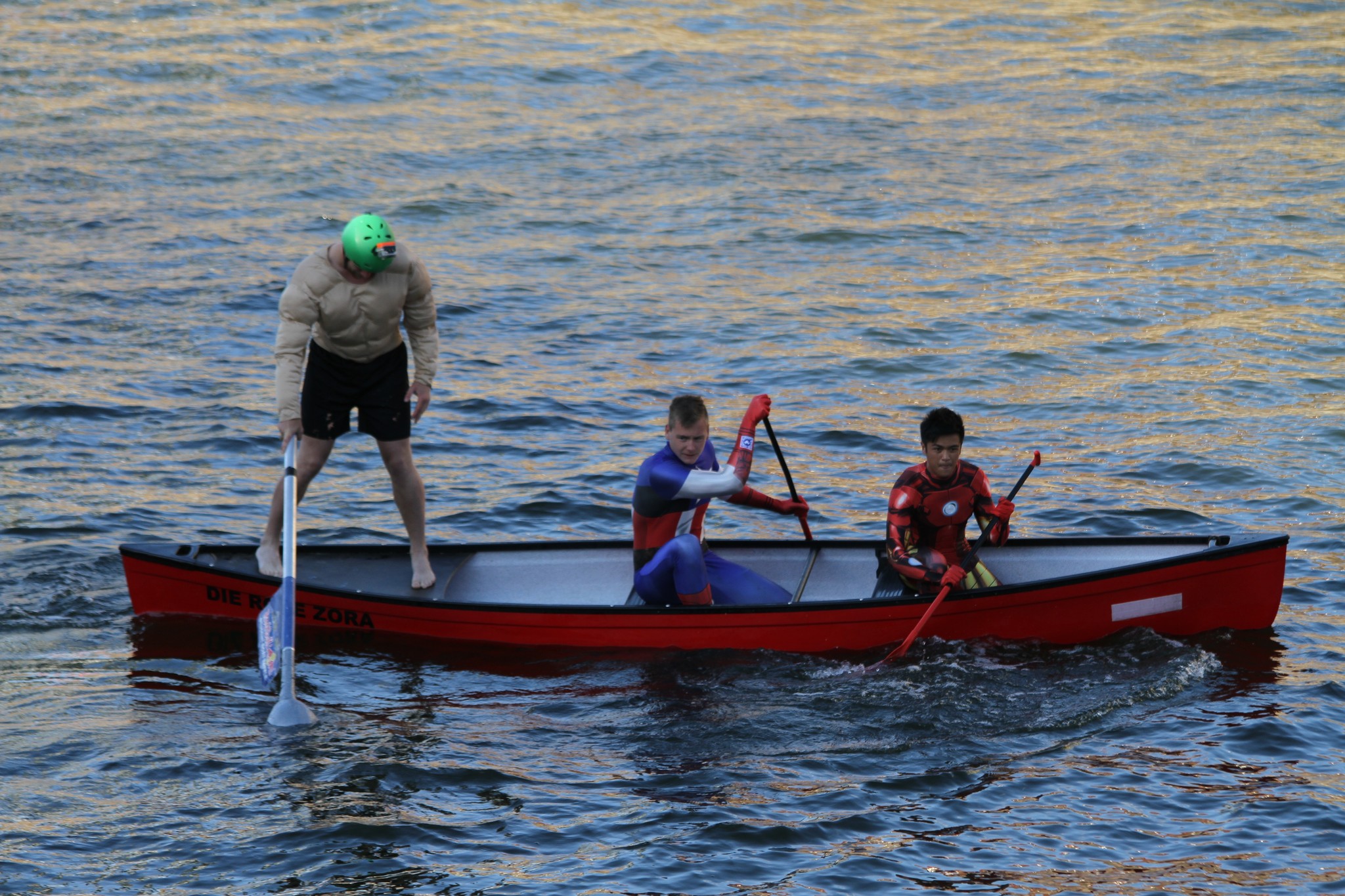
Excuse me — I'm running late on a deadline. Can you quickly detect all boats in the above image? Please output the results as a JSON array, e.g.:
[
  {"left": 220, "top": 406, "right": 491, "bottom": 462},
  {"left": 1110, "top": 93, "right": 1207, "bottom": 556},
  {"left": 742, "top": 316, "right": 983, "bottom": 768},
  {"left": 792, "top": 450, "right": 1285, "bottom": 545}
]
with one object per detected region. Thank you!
[{"left": 117, "top": 529, "right": 1292, "bottom": 653}]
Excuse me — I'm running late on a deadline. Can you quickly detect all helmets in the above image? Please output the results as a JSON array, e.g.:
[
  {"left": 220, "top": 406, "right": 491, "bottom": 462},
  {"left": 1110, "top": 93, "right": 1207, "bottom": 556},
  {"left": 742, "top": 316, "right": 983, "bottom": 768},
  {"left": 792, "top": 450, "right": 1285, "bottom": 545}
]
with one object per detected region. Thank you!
[{"left": 341, "top": 212, "right": 397, "bottom": 273}]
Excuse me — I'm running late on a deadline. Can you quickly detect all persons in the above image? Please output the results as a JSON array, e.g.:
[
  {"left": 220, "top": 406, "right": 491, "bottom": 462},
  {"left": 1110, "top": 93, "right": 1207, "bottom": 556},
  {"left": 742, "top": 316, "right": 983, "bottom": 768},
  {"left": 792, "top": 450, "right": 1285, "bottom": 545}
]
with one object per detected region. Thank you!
[
  {"left": 256, "top": 214, "right": 439, "bottom": 590},
  {"left": 630, "top": 394, "right": 810, "bottom": 608},
  {"left": 886, "top": 406, "right": 1014, "bottom": 596}
]
